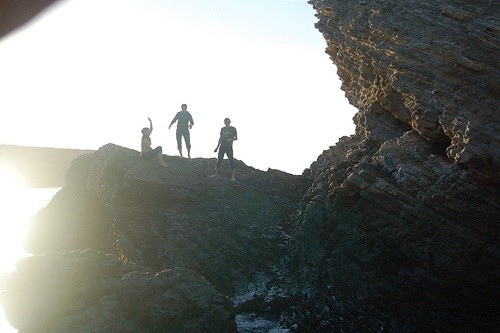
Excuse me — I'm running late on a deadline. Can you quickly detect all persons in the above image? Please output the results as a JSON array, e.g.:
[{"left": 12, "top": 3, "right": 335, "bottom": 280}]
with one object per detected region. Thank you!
[
  {"left": 207, "top": 118, "right": 238, "bottom": 183},
  {"left": 168, "top": 104, "right": 194, "bottom": 159},
  {"left": 140, "top": 117, "right": 162, "bottom": 154}
]
